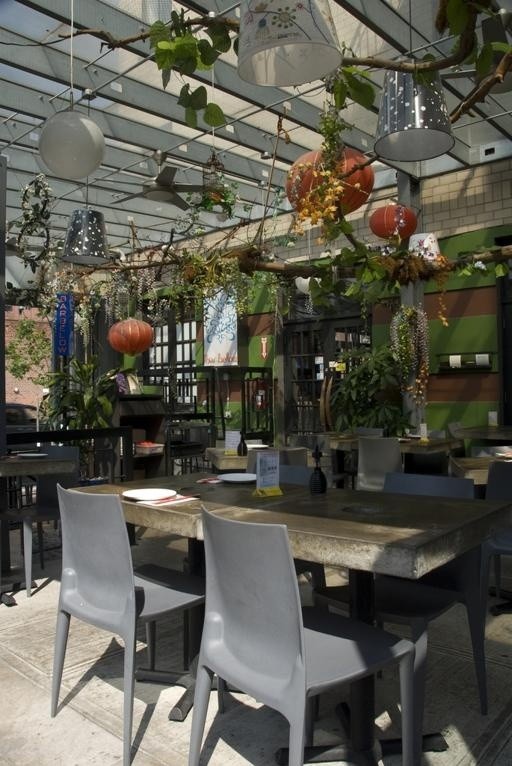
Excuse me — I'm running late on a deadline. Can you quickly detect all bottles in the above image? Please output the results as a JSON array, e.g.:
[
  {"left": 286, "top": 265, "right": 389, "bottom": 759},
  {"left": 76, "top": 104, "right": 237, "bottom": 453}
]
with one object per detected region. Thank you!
[
  {"left": 309, "top": 445, "right": 326, "bottom": 496},
  {"left": 237, "top": 430, "right": 248, "bottom": 457}
]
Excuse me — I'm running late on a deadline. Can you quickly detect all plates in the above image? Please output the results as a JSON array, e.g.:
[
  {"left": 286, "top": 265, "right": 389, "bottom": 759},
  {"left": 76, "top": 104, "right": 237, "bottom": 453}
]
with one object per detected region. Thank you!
[
  {"left": 216, "top": 473, "right": 259, "bottom": 484},
  {"left": 397, "top": 434, "right": 421, "bottom": 443},
  {"left": 18, "top": 453, "right": 48, "bottom": 458},
  {"left": 121, "top": 487, "right": 177, "bottom": 501}
]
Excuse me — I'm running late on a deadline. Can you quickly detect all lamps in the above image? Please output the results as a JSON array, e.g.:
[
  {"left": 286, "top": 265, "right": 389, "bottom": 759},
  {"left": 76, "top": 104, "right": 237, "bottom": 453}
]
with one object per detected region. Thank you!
[
  {"left": 374, "top": 0, "right": 455, "bottom": 162},
  {"left": 294, "top": 219, "right": 322, "bottom": 294},
  {"left": 237, "top": 0, "right": 344, "bottom": 87},
  {"left": 61, "top": 89, "right": 111, "bottom": 266},
  {"left": 408, "top": 160, "right": 441, "bottom": 264},
  {"left": 40, "top": 0, "right": 106, "bottom": 179}
]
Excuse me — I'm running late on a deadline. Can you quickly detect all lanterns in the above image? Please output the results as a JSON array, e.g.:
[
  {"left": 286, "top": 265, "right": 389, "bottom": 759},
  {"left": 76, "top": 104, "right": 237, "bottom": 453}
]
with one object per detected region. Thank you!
[
  {"left": 108, "top": 317, "right": 153, "bottom": 356},
  {"left": 286, "top": 146, "right": 374, "bottom": 216},
  {"left": 369, "top": 205, "right": 417, "bottom": 239}
]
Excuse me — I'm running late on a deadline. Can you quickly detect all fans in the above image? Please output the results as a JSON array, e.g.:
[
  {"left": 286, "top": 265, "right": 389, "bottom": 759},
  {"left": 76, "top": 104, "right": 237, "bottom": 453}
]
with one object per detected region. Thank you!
[{"left": 94, "top": 149, "right": 204, "bottom": 211}]
